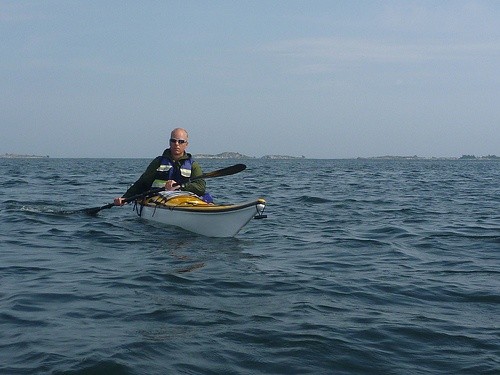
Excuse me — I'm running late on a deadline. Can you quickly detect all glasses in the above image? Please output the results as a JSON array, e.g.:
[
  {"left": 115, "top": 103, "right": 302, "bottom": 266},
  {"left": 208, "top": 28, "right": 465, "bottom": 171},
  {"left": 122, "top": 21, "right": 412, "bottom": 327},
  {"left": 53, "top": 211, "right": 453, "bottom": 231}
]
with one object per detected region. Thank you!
[{"left": 169, "top": 138, "right": 188, "bottom": 144}]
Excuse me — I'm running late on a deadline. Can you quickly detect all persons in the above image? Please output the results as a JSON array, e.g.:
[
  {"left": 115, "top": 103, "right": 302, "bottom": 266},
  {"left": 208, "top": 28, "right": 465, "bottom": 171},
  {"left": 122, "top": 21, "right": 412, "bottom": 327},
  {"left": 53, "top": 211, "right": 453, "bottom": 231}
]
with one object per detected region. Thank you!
[{"left": 114, "top": 128, "right": 206, "bottom": 205}]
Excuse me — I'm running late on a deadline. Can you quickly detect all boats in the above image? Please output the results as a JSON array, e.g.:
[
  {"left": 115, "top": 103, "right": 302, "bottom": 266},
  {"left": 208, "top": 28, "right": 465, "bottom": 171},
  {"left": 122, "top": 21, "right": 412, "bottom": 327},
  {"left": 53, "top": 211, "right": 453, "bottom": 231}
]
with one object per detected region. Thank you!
[{"left": 134, "top": 194, "right": 267, "bottom": 238}]
